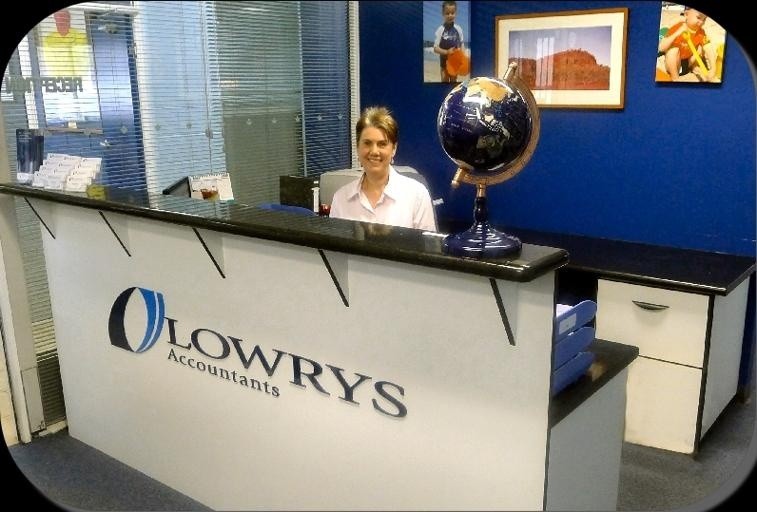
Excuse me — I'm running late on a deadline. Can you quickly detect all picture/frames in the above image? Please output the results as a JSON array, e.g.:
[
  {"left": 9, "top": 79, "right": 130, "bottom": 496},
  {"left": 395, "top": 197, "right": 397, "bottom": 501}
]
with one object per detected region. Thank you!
[{"left": 494, "top": 6, "right": 628, "bottom": 110}]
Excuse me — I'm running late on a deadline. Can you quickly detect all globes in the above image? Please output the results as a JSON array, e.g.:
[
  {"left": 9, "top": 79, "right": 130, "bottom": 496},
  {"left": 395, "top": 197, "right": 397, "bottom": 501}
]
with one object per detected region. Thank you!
[{"left": 437, "top": 61, "right": 541, "bottom": 258}]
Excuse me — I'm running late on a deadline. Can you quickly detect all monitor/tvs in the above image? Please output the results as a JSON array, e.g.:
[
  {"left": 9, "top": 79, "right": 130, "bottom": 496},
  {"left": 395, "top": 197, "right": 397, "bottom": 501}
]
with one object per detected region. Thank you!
[{"left": 163, "top": 176, "right": 191, "bottom": 198}]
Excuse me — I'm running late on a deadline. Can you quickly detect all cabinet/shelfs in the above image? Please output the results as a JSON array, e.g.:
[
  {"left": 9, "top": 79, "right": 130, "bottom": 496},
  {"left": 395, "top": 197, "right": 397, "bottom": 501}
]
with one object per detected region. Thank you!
[{"left": 439, "top": 221, "right": 757, "bottom": 459}]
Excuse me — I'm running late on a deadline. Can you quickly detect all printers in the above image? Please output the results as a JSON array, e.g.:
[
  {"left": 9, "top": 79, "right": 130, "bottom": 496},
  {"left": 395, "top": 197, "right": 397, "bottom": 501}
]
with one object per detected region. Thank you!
[{"left": 320, "top": 165, "right": 444, "bottom": 210}]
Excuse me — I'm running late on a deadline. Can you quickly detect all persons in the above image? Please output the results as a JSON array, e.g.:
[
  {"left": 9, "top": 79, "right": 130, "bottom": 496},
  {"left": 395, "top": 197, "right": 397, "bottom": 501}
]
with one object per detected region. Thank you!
[
  {"left": 327, "top": 103, "right": 440, "bottom": 233},
  {"left": 657, "top": 7, "right": 716, "bottom": 82},
  {"left": 432, "top": 1, "right": 465, "bottom": 83},
  {"left": 328, "top": 216, "right": 443, "bottom": 260}
]
